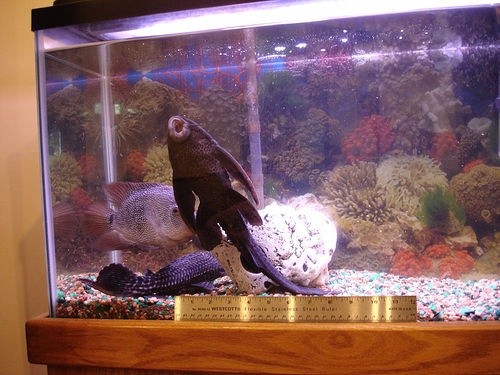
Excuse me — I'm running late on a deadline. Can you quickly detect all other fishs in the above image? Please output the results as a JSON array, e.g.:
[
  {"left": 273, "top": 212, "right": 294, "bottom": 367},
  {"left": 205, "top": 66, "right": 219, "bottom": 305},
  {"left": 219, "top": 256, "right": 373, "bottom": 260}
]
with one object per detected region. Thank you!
[
  {"left": 166, "top": 114, "right": 332, "bottom": 296},
  {"left": 82, "top": 181, "right": 197, "bottom": 253},
  {"left": 76, "top": 247, "right": 229, "bottom": 298}
]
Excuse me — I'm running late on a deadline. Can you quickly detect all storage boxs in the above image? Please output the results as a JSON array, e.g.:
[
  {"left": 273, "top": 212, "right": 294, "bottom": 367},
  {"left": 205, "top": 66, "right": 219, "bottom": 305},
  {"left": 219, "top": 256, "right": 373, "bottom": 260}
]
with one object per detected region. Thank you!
[{"left": 25, "top": 0, "right": 499, "bottom": 374}]
